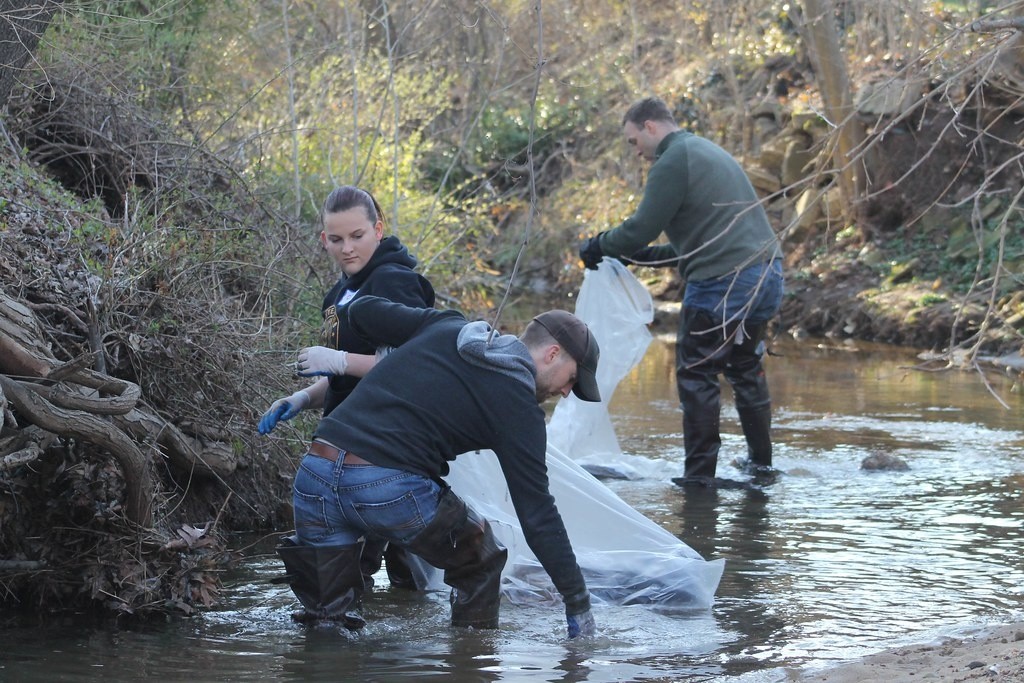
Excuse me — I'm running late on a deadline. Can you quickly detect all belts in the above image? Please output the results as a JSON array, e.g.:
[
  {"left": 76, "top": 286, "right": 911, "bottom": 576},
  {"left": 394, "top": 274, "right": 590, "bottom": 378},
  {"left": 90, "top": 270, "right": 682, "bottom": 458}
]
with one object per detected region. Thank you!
[{"left": 308, "top": 441, "right": 375, "bottom": 465}]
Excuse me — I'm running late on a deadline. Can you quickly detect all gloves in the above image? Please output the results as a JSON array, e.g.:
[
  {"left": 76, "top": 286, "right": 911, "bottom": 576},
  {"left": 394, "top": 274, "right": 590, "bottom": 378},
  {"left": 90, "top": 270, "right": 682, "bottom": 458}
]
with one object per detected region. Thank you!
[
  {"left": 258, "top": 390, "right": 310, "bottom": 434},
  {"left": 297, "top": 346, "right": 348, "bottom": 377},
  {"left": 566, "top": 611, "right": 596, "bottom": 638},
  {"left": 579, "top": 231, "right": 604, "bottom": 270},
  {"left": 612, "top": 257, "right": 630, "bottom": 275}
]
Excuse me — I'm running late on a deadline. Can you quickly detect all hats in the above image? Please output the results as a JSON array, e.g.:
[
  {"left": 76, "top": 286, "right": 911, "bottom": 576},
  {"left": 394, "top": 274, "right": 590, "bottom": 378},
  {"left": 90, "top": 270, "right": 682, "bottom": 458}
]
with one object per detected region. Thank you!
[{"left": 533, "top": 309, "right": 603, "bottom": 402}]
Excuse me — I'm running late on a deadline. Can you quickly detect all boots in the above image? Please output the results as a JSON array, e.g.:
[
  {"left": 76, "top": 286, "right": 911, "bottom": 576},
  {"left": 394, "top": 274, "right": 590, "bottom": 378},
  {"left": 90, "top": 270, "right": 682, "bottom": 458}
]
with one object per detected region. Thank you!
[
  {"left": 407, "top": 486, "right": 508, "bottom": 629},
  {"left": 360, "top": 540, "right": 437, "bottom": 590},
  {"left": 676, "top": 305, "right": 773, "bottom": 476},
  {"left": 274, "top": 542, "right": 365, "bottom": 615}
]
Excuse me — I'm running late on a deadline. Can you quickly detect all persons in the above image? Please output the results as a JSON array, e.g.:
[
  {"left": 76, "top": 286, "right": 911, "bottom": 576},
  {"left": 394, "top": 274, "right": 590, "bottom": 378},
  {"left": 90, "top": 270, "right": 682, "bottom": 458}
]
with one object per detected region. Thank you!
[
  {"left": 275, "top": 293, "right": 602, "bottom": 638},
  {"left": 580, "top": 96, "right": 785, "bottom": 482},
  {"left": 257, "top": 186, "right": 435, "bottom": 593}
]
